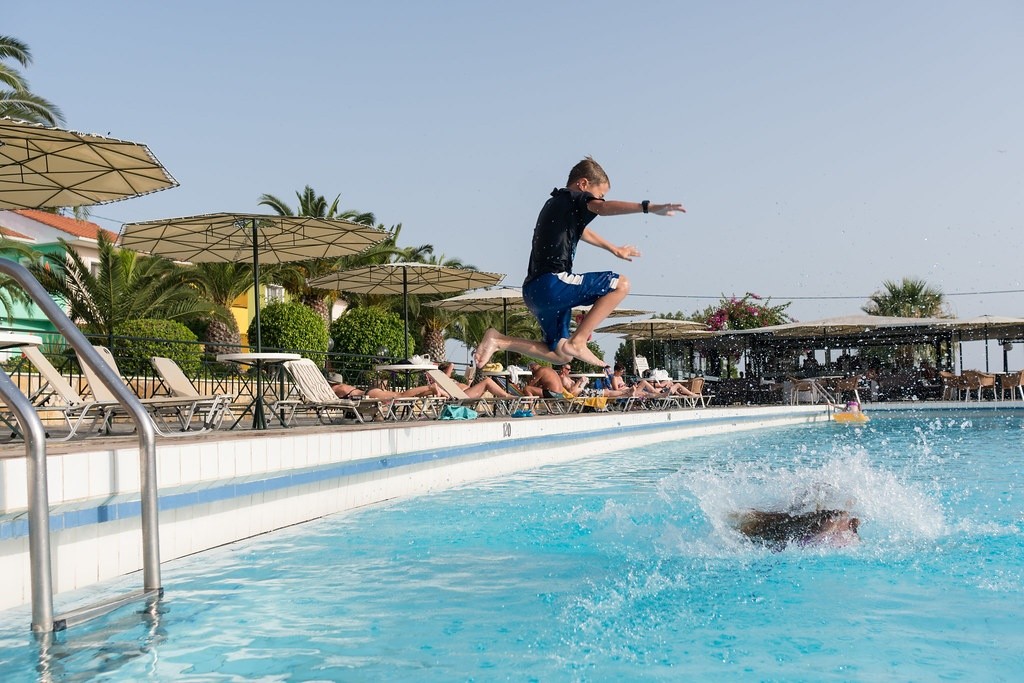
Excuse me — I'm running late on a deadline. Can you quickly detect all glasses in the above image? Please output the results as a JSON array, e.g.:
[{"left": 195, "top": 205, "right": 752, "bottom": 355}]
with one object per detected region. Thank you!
[{"left": 563, "top": 367, "right": 571, "bottom": 372}]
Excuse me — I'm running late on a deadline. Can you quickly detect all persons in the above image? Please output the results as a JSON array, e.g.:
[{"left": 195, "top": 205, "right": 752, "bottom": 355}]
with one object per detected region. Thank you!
[
  {"left": 474, "top": 154, "right": 687, "bottom": 371},
  {"left": 327, "top": 381, "right": 451, "bottom": 402},
  {"left": 559, "top": 363, "right": 640, "bottom": 399},
  {"left": 524, "top": 363, "right": 565, "bottom": 415},
  {"left": 642, "top": 368, "right": 703, "bottom": 399},
  {"left": 611, "top": 363, "right": 673, "bottom": 399},
  {"left": 734, "top": 346, "right": 947, "bottom": 413},
  {"left": 438, "top": 362, "right": 519, "bottom": 401}
]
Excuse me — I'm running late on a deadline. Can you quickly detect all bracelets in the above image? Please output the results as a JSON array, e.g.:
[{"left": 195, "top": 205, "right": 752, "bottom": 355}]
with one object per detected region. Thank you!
[{"left": 642, "top": 201, "right": 651, "bottom": 214}]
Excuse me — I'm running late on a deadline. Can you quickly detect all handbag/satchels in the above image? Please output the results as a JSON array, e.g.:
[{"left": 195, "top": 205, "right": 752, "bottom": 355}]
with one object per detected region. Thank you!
[{"left": 441, "top": 404, "right": 478, "bottom": 421}]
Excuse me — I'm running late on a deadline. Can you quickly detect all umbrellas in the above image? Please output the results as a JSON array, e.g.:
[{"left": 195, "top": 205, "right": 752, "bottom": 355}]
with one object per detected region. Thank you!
[
  {"left": 567, "top": 304, "right": 656, "bottom": 373},
  {"left": 422, "top": 287, "right": 534, "bottom": 419},
  {"left": 604, "top": 313, "right": 1024, "bottom": 382},
  {"left": 305, "top": 264, "right": 508, "bottom": 422},
  {"left": 110, "top": 211, "right": 396, "bottom": 433},
  {"left": 1, "top": 113, "right": 181, "bottom": 214}
]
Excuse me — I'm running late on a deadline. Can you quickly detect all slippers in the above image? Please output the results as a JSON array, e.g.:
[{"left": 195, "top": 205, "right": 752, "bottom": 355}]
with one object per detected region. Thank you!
[
  {"left": 511, "top": 409, "right": 528, "bottom": 418},
  {"left": 522, "top": 410, "right": 533, "bottom": 417}
]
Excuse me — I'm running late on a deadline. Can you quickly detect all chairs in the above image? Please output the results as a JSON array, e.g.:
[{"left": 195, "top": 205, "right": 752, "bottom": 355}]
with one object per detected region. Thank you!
[
  {"left": 485, "top": 381, "right": 718, "bottom": 412},
  {"left": 999, "top": 369, "right": 1024, "bottom": 400},
  {"left": 424, "top": 370, "right": 519, "bottom": 417},
  {"left": 344, "top": 393, "right": 448, "bottom": 421},
  {"left": 960, "top": 368, "right": 997, "bottom": 402},
  {"left": 939, "top": 370, "right": 966, "bottom": 401},
  {"left": 20, "top": 345, "right": 122, "bottom": 443},
  {"left": 833, "top": 374, "right": 864, "bottom": 405},
  {"left": 152, "top": 356, "right": 246, "bottom": 431},
  {"left": 283, "top": 357, "right": 368, "bottom": 425},
  {"left": 73, "top": 345, "right": 220, "bottom": 437},
  {"left": 787, "top": 374, "right": 815, "bottom": 405}
]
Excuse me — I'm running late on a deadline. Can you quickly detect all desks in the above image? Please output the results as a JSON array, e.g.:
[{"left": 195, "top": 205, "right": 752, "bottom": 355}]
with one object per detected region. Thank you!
[
  {"left": 376, "top": 363, "right": 438, "bottom": 419},
  {"left": 213, "top": 353, "right": 303, "bottom": 429},
  {"left": 0, "top": 332, "right": 43, "bottom": 447}
]
